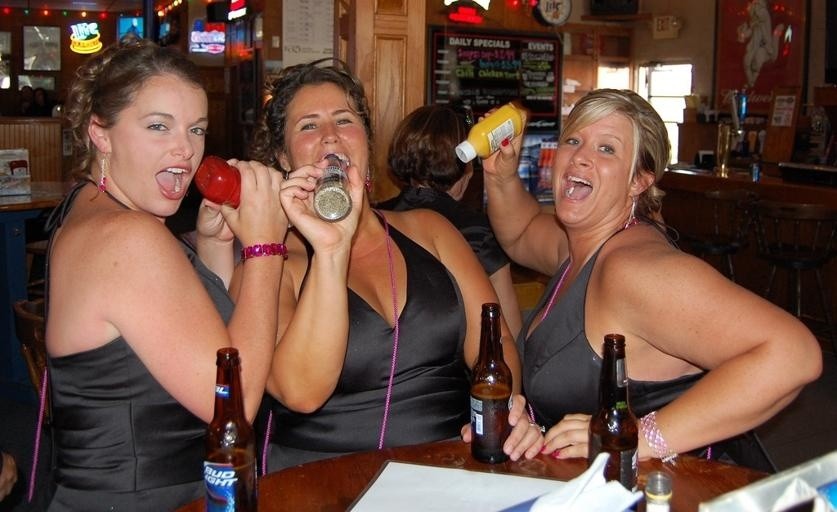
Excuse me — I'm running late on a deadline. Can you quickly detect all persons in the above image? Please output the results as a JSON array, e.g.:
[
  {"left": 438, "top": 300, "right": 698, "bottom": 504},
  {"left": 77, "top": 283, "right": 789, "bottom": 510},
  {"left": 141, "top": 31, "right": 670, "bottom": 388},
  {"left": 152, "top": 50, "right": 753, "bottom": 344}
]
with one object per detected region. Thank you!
[
  {"left": 0, "top": 303, "right": 37, "bottom": 512},
  {"left": 370, "top": 101, "right": 524, "bottom": 341},
  {"left": 43, "top": 36, "right": 289, "bottom": 512},
  {"left": 475, "top": 89, "right": 825, "bottom": 462},
  {"left": 229, "top": 58, "right": 547, "bottom": 463}
]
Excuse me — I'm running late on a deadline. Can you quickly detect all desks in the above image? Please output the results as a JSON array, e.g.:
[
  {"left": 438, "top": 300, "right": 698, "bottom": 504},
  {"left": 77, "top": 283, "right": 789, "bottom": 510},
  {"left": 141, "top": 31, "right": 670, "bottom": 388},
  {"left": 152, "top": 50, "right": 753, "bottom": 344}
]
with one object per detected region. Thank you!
[
  {"left": 0, "top": 182, "right": 78, "bottom": 484},
  {"left": 177, "top": 438, "right": 836, "bottom": 512}
]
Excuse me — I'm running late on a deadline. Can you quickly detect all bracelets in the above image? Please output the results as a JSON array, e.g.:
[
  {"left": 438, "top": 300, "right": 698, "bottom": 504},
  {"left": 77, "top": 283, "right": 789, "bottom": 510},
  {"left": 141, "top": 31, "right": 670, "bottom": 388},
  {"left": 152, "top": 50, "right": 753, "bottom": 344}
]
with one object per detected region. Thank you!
[
  {"left": 234, "top": 241, "right": 291, "bottom": 263},
  {"left": 641, "top": 412, "right": 677, "bottom": 465}
]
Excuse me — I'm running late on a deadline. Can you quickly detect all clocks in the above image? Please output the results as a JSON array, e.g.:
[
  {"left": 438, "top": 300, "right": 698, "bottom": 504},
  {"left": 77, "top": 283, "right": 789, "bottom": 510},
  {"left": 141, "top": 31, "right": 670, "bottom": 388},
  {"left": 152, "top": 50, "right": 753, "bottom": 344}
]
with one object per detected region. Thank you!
[{"left": 534, "top": 0, "right": 573, "bottom": 28}]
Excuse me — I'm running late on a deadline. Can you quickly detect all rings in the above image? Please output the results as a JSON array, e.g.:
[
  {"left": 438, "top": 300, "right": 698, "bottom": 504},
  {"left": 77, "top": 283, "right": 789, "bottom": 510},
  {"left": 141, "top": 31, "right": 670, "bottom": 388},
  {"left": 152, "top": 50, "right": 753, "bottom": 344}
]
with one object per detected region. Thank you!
[{"left": 530, "top": 422, "right": 546, "bottom": 434}]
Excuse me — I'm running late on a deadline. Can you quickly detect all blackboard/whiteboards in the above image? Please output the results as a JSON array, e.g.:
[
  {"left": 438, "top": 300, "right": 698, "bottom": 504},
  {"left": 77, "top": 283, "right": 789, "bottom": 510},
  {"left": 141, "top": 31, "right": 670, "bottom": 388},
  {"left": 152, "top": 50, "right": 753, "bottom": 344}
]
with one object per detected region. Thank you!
[{"left": 426, "top": 23, "right": 564, "bottom": 202}]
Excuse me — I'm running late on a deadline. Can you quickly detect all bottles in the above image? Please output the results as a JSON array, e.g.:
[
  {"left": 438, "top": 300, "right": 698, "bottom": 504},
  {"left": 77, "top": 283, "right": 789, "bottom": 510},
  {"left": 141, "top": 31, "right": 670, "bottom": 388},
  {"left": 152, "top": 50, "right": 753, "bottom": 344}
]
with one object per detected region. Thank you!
[
  {"left": 588, "top": 333, "right": 639, "bottom": 487},
  {"left": 313, "top": 154, "right": 353, "bottom": 223},
  {"left": 749, "top": 163, "right": 762, "bottom": 184},
  {"left": 759, "top": 128, "right": 766, "bottom": 153},
  {"left": 735, "top": 122, "right": 746, "bottom": 156},
  {"left": 729, "top": 94, "right": 738, "bottom": 118},
  {"left": 455, "top": 102, "right": 523, "bottom": 165},
  {"left": 203, "top": 346, "right": 258, "bottom": 512},
  {"left": 730, "top": 128, "right": 736, "bottom": 155},
  {"left": 636, "top": 471, "right": 677, "bottom": 512},
  {"left": 738, "top": 95, "right": 747, "bottom": 122},
  {"left": 194, "top": 154, "right": 241, "bottom": 207},
  {"left": 469, "top": 302, "right": 514, "bottom": 465},
  {"left": 747, "top": 127, "right": 757, "bottom": 152}
]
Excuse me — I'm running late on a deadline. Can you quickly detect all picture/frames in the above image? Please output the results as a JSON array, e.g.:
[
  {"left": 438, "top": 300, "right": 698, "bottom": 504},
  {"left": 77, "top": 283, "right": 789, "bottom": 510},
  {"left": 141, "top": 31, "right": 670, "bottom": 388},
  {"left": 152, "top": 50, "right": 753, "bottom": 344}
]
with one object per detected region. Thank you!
[{"left": 711, "top": 0, "right": 812, "bottom": 120}]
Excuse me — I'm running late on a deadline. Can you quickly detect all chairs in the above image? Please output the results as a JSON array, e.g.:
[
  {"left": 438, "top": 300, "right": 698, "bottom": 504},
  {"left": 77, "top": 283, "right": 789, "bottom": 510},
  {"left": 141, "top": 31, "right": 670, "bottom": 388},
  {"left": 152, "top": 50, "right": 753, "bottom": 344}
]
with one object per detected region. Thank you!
[
  {"left": 682, "top": 188, "right": 758, "bottom": 285},
  {"left": 749, "top": 200, "right": 836, "bottom": 327},
  {"left": 0, "top": 117, "right": 64, "bottom": 181},
  {"left": 14, "top": 299, "right": 58, "bottom": 435}
]
